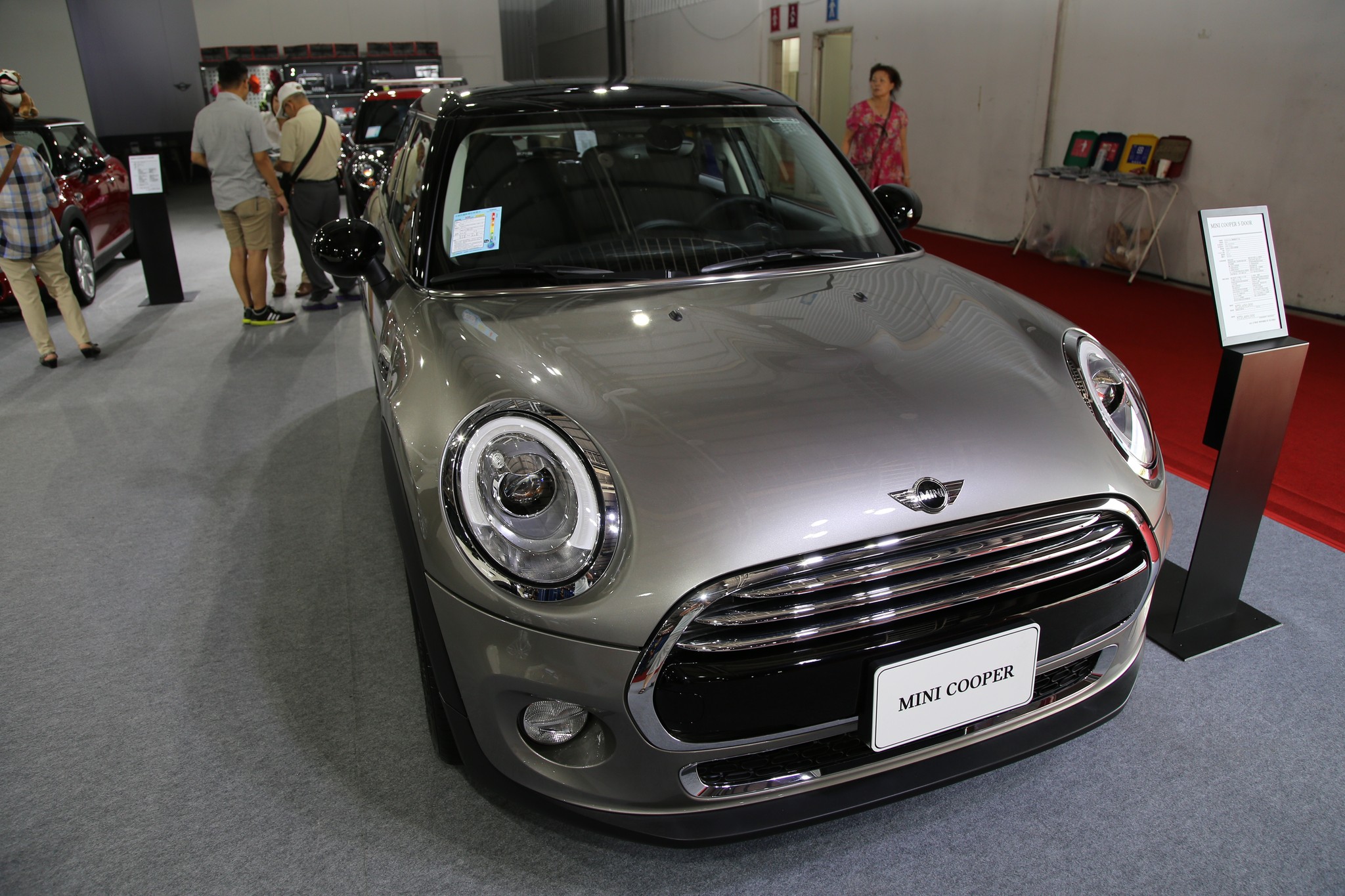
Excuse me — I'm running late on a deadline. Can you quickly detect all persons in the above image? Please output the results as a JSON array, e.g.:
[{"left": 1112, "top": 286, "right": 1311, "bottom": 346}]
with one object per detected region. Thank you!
[
  {"left": 386, "top": 129, "right": 431, "bottom": 237},
  {"left": 841, "top": 63, "right": 911, "bottom": 193},
  {"left": 0, "top": 109, "right": 101, "bottom": 368},
  {"left": 190, "top": 59, "right": 362, "bottom": 325}
]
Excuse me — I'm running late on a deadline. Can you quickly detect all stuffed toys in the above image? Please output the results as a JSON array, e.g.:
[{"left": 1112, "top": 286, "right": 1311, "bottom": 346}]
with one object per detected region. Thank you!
[{"left": 0, "top": 69, "right": 39, "bottom": 121}]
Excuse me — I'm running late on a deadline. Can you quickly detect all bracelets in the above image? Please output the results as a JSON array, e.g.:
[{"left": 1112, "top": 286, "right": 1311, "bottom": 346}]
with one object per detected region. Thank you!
[{"left": 275, "top": 190, "right": 284, "bottom": 197}]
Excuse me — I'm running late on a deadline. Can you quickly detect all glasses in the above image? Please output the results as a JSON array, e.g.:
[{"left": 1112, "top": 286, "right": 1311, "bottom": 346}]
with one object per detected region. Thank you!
[{"left": 282, "top": 99, "right": 290, "bottom": 118}]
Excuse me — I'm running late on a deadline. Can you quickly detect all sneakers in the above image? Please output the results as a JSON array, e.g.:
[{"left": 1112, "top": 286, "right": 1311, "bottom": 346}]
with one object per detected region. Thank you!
[
  {"left": 251, "top": 306, "right": 296, "bottom": 325},
  {"left": 243, "top": 307, "right": 252, "bottom": 324}
]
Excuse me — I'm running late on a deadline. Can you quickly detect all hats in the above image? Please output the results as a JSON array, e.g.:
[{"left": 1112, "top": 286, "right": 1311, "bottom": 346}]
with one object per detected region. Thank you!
[{"left": 275, "top": 81, "right": 303, "bottom": 118}]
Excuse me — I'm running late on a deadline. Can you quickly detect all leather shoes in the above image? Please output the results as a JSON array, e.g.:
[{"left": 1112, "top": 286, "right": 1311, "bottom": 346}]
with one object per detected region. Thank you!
[
  {"left": 272, "top": 282, "right": 286, "bottom": 297},
  {"left": 295, "top": 282, "right": 310, "bottom": 297}
]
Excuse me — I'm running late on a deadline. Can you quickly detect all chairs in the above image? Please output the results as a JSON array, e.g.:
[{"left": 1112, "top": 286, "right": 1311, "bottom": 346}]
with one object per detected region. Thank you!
[{"left": 466, "top": 138, "right": 738, "bottom": 251}]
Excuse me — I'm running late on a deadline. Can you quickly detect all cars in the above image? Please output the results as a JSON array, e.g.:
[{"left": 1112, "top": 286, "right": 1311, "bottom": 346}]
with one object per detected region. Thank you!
[
  {"left": 0, "top": 118, "right": 140, "bottom": 314},
  {"left": 302, "top": 78, "right": 1172, "bottom": 846},
  {"left": 339, "top": 87, "right": 433, "bottom": 223},
  {"left": 304, "top": 93, "right": 371, "bottom": 191}
]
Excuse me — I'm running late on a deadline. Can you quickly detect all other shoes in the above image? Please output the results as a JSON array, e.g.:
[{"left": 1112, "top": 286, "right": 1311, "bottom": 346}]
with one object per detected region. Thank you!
[
  {"left": 302, "top": 290, "right": 339, "bottom": 311},
  {"left": 332, "top": 282, "right": 362, "bottom": 300}
]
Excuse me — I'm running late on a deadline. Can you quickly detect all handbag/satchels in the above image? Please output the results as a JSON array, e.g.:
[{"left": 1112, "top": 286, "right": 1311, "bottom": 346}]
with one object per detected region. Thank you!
[
  {"left": 857, "top": 165, "right": 873, "bottom": 185},
  {"left": 279, "top": 171, "right": 293, "bottom": 211}
]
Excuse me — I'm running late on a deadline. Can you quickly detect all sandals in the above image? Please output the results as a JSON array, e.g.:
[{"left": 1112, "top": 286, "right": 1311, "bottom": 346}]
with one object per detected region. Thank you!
[
  {"left": 80, "top": 342, "right": 102, "bottom": 359},
  {"left": 39, "top": 351, "right": 58, "bottom": 369}
]
